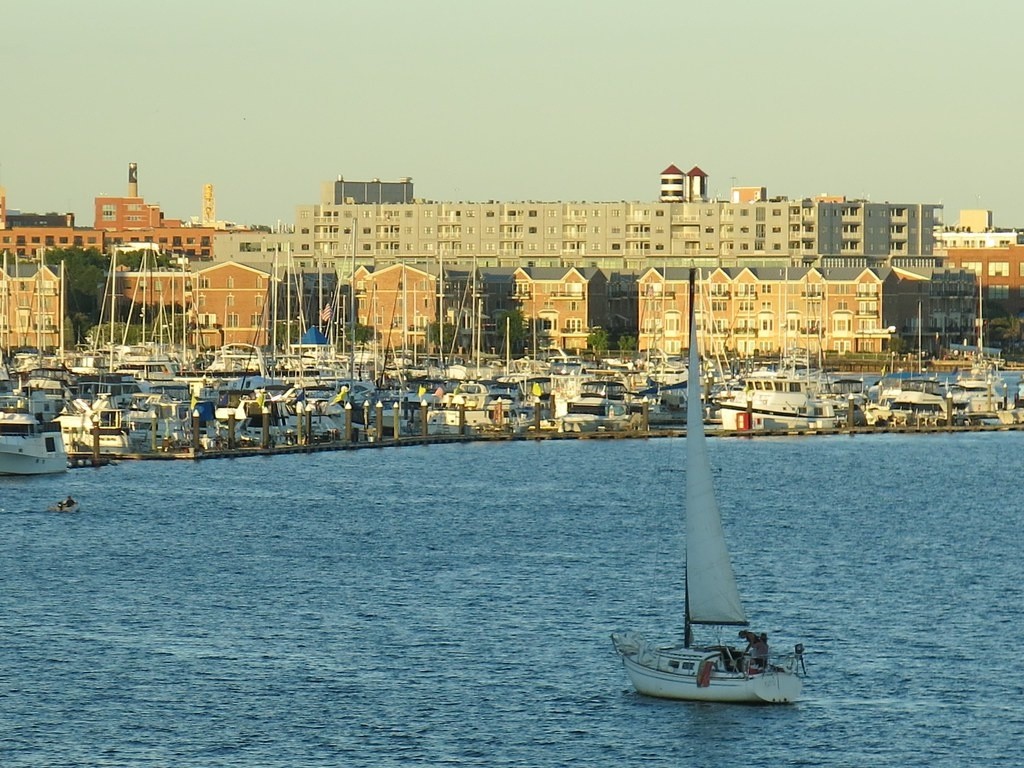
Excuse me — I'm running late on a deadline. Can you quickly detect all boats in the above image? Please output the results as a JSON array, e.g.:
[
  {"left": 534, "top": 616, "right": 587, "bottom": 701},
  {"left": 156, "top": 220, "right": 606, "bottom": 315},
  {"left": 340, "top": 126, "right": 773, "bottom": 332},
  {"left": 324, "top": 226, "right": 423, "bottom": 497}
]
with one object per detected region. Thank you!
[{"left": 47, "top": 495, "right": 81, "bottom": 513}]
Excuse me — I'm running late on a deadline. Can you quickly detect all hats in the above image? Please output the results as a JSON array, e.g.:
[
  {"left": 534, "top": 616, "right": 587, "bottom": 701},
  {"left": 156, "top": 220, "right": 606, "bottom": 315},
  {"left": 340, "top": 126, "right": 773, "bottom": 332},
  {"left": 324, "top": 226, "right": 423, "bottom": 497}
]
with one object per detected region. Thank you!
[
  {"left": 738, "top": 629, "right": 747, "bottom": 638},
  {"left": 760, "top": 633, "right": 770, "bottom": 639}
]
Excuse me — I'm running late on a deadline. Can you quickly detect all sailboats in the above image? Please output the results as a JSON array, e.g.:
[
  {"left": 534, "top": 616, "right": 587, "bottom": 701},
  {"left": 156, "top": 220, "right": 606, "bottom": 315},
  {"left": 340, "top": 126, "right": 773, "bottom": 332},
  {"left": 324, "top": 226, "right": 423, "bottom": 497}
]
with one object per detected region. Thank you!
[
  {"left": 232, "top": 219, "right": 1024, "bottom": 455},
  {"left": 608, "top": 267, "right": 809, "bottom": 706},
  {"left": 0, "top": 249, "right": 290, "bottom": 477}
]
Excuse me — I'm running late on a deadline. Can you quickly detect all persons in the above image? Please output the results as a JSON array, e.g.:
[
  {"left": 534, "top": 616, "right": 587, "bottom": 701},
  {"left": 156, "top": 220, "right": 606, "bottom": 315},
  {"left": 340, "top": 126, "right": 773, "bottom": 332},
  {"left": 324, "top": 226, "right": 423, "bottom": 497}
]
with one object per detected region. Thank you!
[
  {"left": 739, "top": 630, "right": 768, "bottom": 669},
  {"left": 407, "top": 420, "right": 415, "bottom": 437},
  {"left": 65, "top": 495, "right": 74, "bottom": 507}
]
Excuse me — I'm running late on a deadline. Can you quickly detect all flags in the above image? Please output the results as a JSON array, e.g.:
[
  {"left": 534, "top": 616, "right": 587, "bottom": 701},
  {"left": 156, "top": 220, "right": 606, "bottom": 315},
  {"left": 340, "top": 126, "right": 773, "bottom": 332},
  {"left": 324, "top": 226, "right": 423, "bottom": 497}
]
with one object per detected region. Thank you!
[
  {"left": 190, "top": 394, "right": 197, "bottom": 410},
  {"left": 321, "top": 303, "right": 333, "bottom": 322},
  {"left": 333, "top": 389, "right": 345, "bottom": 403},
  {"left": 256, "top": 391, "right": 263, "bottom": 406},
  {"left": 297, "top": 392, "right": 304, "bottom": 401},
  {"left": 533, "top": 382, "right": 544, "bottom": 397},
  {"left": 419, "top": 385, "right": 426, "bottom": 397},
  {"left": 219, "top": 394, "right": 228, "bottom": 408}
]
